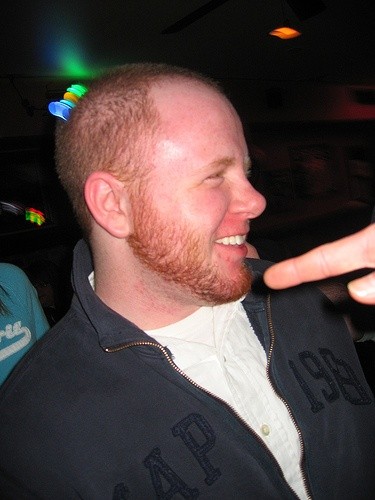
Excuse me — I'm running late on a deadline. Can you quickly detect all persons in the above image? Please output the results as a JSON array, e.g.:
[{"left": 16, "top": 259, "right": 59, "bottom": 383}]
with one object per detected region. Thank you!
[
  {"left": 263, "top": 221, "right": 375, "bottom": 305},
  {"left": 0, "top": 62, "right": 375, "bottom": 500},
  {"left": 0, "top": 262, "right": 50, "bottom": 387}
]
